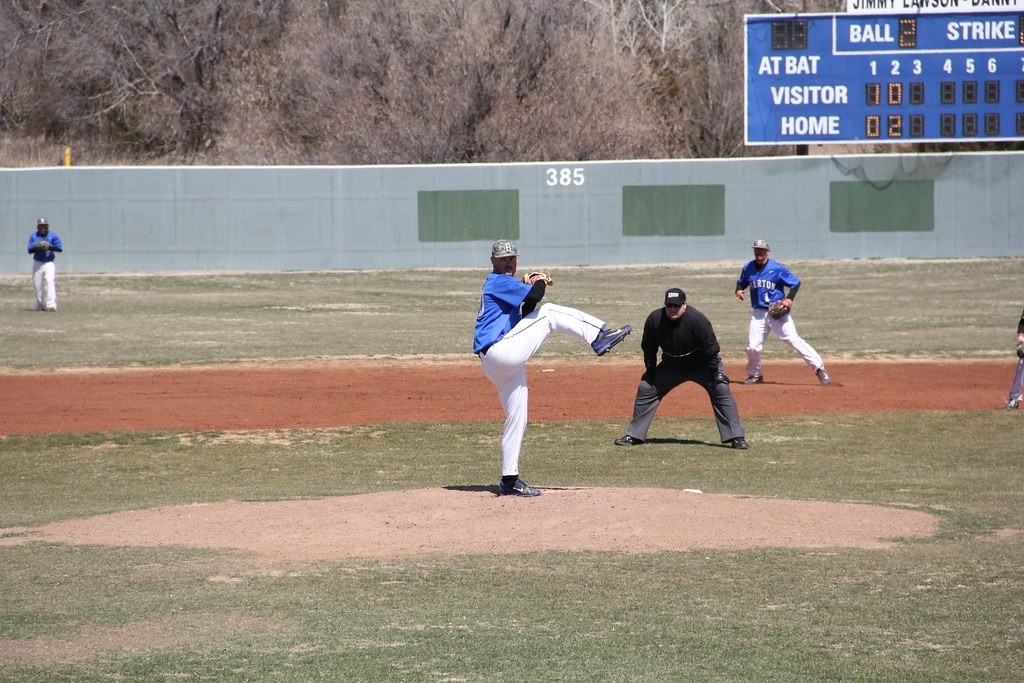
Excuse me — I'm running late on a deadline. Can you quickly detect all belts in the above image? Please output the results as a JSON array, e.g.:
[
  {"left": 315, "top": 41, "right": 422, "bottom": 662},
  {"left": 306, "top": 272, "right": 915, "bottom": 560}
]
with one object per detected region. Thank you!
[{"left": 479, "top": 342, "right": 494, "bottom": 359}]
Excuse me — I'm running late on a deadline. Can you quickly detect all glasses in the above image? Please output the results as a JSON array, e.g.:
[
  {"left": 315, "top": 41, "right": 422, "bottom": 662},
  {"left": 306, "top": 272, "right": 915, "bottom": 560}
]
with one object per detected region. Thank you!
[{"left": 666, "top": 304, "right": 681, "bottom": 308}]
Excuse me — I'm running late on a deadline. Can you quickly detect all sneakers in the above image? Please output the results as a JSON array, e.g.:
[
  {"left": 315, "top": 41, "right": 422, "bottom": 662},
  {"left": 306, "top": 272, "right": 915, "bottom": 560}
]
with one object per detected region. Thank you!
[
  {"left": 732, "top": 439, "right": 749, "bottom": 448},
  {"left": 591, "top": 324, "right": 634, "bottom": 356},
  {"left": 499, "top": 478, "right": 541, "bottom": 497},
  {"left": 614, "top": 435, "right": 644, "bottom": 445}
]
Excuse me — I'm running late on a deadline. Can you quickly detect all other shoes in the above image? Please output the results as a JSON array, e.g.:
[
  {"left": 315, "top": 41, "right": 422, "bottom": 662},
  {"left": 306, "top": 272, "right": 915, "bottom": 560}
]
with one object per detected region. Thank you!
[
  {"left": 1007, "top": 400, "right": 1019, "bottom": 411},
  {"left": 36, "top": 303, "right": 57, "bottom": 312},
  {"left": 741, "top": 375, "right": 763, "bottom": 384},
  {"left": 816, "top": 369, "right": 831, "bottom": 385}
]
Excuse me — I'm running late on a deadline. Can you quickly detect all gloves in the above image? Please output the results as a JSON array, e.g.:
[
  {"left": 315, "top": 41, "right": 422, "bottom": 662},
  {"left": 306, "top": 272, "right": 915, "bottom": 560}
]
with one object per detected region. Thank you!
[
  {"left": 641, "top": 367, "right": 656, "bottom": 386},
  {"left": 711, "top": 368, "right": 729, "bottom": 387}
]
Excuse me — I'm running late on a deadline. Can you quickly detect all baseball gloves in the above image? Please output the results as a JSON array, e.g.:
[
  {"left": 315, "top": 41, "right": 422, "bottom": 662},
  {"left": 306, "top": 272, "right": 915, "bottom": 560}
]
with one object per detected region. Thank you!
[
  {"left": 522, "top": 271, "right": 554, "bottom": 287},
  {"left": 34, "top": 240, "right": 53, "bottom": 251},
  {"left": 768, "top": 300, "right": 791, "bottom": 320}
]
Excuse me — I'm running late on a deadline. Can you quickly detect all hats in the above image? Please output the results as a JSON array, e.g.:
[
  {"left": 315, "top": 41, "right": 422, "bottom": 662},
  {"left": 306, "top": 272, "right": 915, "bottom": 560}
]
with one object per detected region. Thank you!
[
  {"left": 38, "top": 218, "right": 48, "bottom": 225},
  {"left": 492, "top": 239, "right": 519, "bottom": 258},
  {"left": 751, "top": 240, "right": 769, "bottom": 249},
  {"left": 665, "top": 288, "right": 686, "bottom": 306}
]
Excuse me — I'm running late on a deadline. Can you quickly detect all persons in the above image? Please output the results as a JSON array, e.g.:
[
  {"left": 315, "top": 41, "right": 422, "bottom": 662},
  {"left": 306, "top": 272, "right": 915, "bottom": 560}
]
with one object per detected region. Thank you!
[
  {"left": 473, "top": 239, "right": 633, "bottom": 498},
  {"left": 28, "top": 218, "right": 63, "bottom": 312},
  {"left": 614, "top": 288, "right": 749, "bottom": 449},
  {"left": 735, "top": 240, "right": 831, "bottom": 384},
  {"left": 1007, "top": 307, "right": 1024, "bottom": 409}
]
